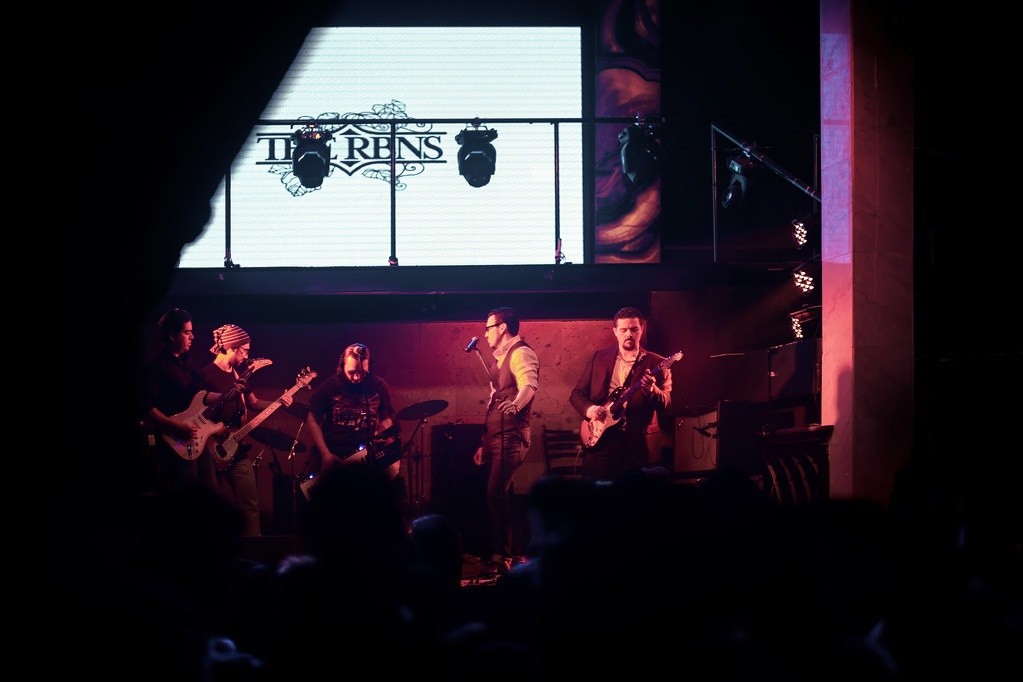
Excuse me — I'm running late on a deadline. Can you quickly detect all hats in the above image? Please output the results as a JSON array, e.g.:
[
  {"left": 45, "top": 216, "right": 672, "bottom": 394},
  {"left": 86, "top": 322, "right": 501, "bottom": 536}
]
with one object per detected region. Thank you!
[{"left": 210, "top": 324, "right": 251, "bottom": 354}]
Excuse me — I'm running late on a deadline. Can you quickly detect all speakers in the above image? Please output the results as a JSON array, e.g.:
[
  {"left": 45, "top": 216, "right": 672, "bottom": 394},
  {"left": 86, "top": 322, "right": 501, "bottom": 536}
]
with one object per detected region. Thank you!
[{"left": 673, "top": 400, "right": 742, "bottom": 477}]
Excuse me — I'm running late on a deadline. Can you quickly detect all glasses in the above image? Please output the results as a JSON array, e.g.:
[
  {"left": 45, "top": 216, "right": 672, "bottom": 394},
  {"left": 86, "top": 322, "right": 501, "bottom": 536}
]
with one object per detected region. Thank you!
[
  {"left": 240, "top": 346, "right": 250, "bottom": 356},
  {"left": 485, "top": 323, "right": 500, "bottom": 331}
]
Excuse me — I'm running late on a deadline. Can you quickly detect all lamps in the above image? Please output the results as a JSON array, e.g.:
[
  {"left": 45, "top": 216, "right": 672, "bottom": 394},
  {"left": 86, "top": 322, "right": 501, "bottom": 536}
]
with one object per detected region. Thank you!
[
  {"left": 289, "top": 124, "right": 333, "bottom": 189},
  {"left": 788, "top": 212, "right": 821, "bottom": 248},
  {"left": 790, "top": 305, "right": 823, "bottom": 340},
  {"left": 456, "top": 124, "right": 498, "bottom": 188},
  {"left": 791, "top": 260, "right": 821, "bottom": 295},
  {"left": 618, "top": 122, "right": 664, "bottom": 185},
  {"left": 722, "top": 156, "right": 775, "bottom": 209}
]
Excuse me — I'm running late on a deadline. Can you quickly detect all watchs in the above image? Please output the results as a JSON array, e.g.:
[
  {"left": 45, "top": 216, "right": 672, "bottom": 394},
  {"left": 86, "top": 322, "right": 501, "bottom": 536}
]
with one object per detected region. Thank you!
[{"left": 513, "top": 405, "right": 520, "bottom": 414}]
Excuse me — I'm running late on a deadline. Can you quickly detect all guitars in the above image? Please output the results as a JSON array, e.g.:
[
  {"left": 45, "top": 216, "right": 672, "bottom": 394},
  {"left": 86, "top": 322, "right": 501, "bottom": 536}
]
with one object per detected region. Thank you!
[
  {"left": 578, "top": 349, "right": 684, "bottom": 455},
  {"left": 203, "top": 366, "right": 320, "bottom": 472},
  {"left": 159, "top": 356, "right": 273, "bottom": 464}
]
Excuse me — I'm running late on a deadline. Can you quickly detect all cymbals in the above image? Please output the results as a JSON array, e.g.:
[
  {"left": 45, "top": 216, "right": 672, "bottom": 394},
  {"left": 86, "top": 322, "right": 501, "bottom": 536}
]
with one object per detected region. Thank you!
[
  {"left": 248, "top": 426, "right": 308, "bottom": 454},
  {"left": 281, "top": 402, "right": 324, "bottom": 426},
  {"left": 396, "top": 399, "right": 449, "bottom": 420}
]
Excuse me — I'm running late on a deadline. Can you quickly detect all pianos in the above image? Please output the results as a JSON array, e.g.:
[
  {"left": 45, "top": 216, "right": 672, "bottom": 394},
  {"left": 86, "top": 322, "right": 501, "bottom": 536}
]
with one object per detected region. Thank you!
[{"left": 295, "top": 436, "right": 401, "bottom": 514}]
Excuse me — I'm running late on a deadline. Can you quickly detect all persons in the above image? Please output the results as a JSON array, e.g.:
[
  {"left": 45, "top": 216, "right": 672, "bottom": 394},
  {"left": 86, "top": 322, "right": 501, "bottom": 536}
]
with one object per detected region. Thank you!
[
  {"left": 196, "top": 324, "right": 294, "bottom": 540},
  {"left": 474, "top": 307, "right": 540, "bottom": 568},
  {"left": 131, "top": 306, "right": 247, "bottom": 503},
  {"left": 568, "top": 306, "right": 673, "bottom": 479},
  {"left": 305, "top": 343, "right": 398, "bottom": 498}
]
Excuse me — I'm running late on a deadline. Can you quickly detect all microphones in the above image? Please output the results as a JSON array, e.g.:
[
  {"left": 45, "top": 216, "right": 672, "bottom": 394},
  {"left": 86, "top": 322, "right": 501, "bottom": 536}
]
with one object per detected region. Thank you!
[
  {"left": 466, "top": 336, "right": 480, "bottom": 353},
  {"left": 693, "top": 426, "right": 711, "bottom": 437}
]
